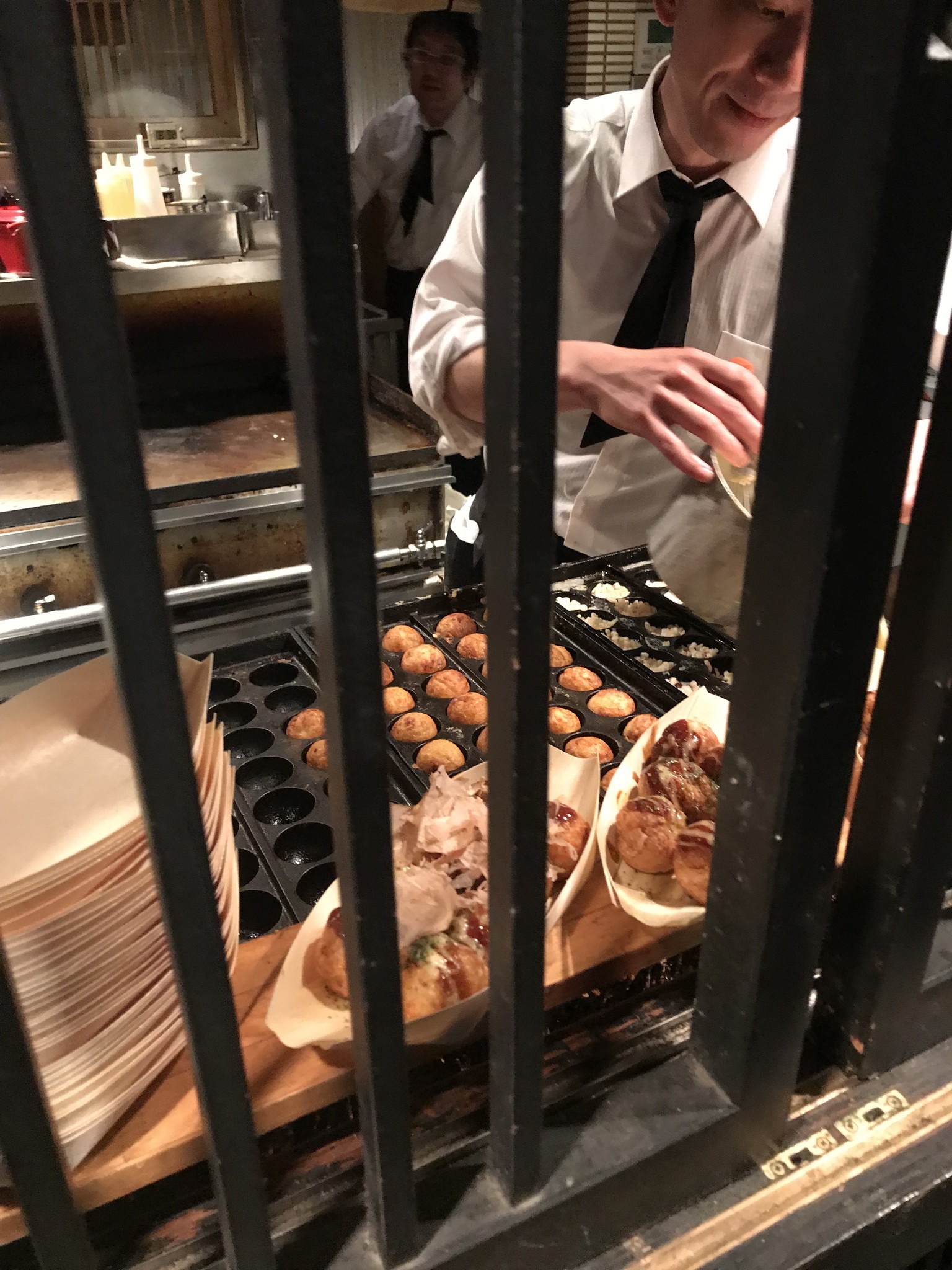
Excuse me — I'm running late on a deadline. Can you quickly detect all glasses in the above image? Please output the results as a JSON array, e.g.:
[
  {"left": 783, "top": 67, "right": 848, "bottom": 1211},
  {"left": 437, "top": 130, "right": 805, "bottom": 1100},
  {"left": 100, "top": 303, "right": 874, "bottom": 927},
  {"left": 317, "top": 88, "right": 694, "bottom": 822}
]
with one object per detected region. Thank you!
[{"left": 398, "top": 45, "right": 468, "bottom": 68}]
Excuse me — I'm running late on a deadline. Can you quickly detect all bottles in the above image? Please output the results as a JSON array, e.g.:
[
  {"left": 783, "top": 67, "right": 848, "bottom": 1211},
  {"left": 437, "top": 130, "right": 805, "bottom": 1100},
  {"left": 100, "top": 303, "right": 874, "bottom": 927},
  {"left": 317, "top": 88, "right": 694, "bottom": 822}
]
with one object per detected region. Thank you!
[
  {"left": 96, "top": 134, "right": 166, "bottom": 220},
  {"left": 177, "top": 153, "right": 204, "bottom": 200},
  {"left": 166, "top": 200, "right": 206, "bottom": 216}
]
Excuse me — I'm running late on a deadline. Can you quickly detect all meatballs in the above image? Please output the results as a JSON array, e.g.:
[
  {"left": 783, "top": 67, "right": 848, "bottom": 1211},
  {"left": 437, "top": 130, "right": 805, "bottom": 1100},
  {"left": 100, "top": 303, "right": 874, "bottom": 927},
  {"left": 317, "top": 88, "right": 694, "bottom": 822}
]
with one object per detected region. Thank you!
[
  {"left": 288, "top": 612, "right": 657, "bottom": 791},
  {"left": 607, "top": 720, "right": 724, "bottom": 905},
  {"left": 302, "top": 764, "right": 590, "bottom": 1020}
]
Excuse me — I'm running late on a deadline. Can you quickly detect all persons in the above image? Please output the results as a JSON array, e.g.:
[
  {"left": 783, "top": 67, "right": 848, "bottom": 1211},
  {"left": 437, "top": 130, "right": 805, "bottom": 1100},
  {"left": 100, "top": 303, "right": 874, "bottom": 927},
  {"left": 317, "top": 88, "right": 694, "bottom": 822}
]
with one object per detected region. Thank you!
[
  {"left": 350, "top": 10, "right": 484, "bottom": 495},
  {"left": 407, "top": 0, "right": 952, "bottom": 593}
]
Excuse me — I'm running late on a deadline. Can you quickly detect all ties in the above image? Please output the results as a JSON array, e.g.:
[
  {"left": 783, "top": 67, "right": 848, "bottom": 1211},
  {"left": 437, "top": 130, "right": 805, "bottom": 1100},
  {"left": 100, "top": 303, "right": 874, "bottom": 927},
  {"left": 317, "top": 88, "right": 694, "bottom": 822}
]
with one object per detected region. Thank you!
[
  {"left": 576, "top": 171, "right": 732, "bottom": 456},
  {"left": 398, "top": 127, "right": 449, "bottom": 240}
]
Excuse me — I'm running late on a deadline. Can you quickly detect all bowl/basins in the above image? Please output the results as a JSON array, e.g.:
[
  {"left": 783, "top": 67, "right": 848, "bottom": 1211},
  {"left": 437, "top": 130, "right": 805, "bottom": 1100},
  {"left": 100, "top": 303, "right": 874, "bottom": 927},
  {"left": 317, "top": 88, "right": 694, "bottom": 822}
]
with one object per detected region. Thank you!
[
  {"left": 646, "top": 447, "right": 753, "bottom": 626},
  {"left": 0, "top": 206, "right": 30, "bottom": 274},
  {"left": 206, "top": 198, "right": 243, "bottom": 213}
]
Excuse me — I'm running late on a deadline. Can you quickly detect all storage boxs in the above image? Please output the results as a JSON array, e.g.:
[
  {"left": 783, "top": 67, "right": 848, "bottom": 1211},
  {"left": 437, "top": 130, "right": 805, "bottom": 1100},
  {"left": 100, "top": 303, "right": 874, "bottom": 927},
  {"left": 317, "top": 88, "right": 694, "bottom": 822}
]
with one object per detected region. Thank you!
[
  {"left": 264, "top": 748, "right": 602, "bottom": 1058},
  {"left": 0, "top": 637, "right": 240, "bottom": 1194},
  {"left": 595, "top": 684, "right": 734, "bottom": 931}
]
